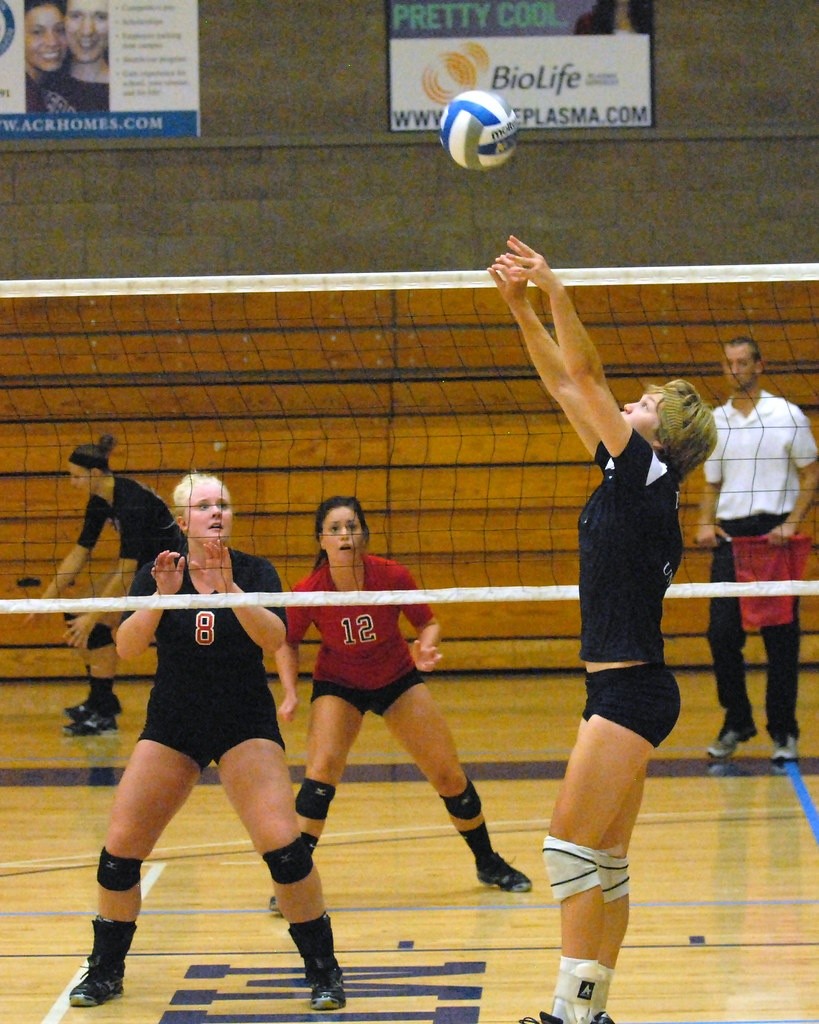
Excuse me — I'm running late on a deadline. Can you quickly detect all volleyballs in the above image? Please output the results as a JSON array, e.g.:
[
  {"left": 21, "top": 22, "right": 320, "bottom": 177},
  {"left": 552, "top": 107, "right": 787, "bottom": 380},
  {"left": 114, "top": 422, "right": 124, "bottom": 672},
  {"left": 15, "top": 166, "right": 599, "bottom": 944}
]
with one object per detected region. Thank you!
[{"left": 437, "top": 89, "right": 521, "bottom": 169}]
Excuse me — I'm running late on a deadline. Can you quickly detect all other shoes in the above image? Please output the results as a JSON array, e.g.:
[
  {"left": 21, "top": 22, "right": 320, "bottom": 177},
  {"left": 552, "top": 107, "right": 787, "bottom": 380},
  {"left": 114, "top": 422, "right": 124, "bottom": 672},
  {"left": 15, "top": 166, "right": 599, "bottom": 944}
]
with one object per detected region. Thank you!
[
  {"left": 590, "top": 1011, "right": 615, "bottom": 1024},
  {"left": 519, "top": 1012, "right": 563, "bottom": 1024}
]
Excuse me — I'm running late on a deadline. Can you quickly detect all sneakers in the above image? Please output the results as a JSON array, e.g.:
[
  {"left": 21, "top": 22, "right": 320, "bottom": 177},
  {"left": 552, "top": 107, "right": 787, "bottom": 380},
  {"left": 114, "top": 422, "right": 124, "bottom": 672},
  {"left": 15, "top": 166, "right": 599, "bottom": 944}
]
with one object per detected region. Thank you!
[
  {"left": 64, "top": 712, "right": 116, "bottom": 736},
  {"left": 303, "top": 957, "right": 346, "bottom": 1009},
  {"left": 708, "top": 723, "right": 757, "bottom": 758},
  {"left": 270, "top": 896, "right": 279, "bottom": 910},
  {"left": 69, "top": 955, "right": 124, "bottom": 1006},
  {"left": 66, "top": 696, "right": 122, "bottom": 721},
  {"left": 475, "top": 853, "right": 532, "bottom": 892},
  {"left": 772, "top": 732, "right": 798, "bottom": 762}
]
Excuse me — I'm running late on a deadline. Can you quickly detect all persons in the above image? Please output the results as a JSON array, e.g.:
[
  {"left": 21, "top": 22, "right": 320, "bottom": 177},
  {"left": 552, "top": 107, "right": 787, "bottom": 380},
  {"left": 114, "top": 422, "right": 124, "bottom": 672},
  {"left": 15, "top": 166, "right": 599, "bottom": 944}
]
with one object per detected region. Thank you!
[
  {"left": 25, "top": 0, "right": 110, "bottom": 114},
  {"left": 574, "top": 0, "right": 654, "bottom": 35},
  {"left": 696, "top": 337, "right": 819, "bottom": 763},
  {"left": 69, "top": 473, "right": 347, "bottom": 1010},
  {"left": 270, "top": 494, "right": 532, "bottom": 918},
  {"left": 21, "top": 435, "right": 188, "bottom": 737},
  {"left": 486, "top": 234, "right": 717, "bottom": 1023}
]
